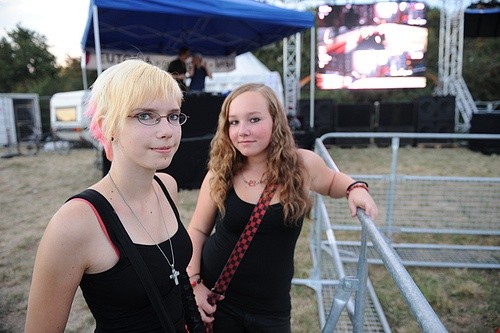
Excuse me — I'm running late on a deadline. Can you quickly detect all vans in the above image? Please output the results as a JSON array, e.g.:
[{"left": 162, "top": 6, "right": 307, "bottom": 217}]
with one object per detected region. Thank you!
[{"left": 49, "top": 89, "right": 86, "bottom": 142}]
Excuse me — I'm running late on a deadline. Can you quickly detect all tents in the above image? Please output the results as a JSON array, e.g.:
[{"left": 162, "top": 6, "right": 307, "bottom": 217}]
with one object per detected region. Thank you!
[{"left": 80, "top": 0, "right": 316, "bottom": 169}]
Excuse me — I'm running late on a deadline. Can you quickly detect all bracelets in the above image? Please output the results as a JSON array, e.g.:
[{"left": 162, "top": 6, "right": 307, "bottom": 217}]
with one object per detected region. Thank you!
[
  {"left": 188, "top": 272, "right": 200, "bottom": 279},
  {"left": 345, "top": 181, "right": 369, "bottom": 199},
  {"left": 191, "top": 279, "right": 203, "bottom": 287}
]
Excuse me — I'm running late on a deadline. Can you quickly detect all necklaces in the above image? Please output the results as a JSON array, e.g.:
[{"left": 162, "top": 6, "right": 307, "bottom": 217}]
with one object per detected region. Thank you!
[
  {"left": 240, "top": 173, "right": 265, "bottom": 186},
  {"left": 108, "top": 172, "right": 180, "bottom": 285}
]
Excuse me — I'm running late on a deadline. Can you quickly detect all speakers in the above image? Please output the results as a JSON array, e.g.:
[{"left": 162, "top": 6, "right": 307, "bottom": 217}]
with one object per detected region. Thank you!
[
  {"left": 294, "top": 95, "right": 456, "bottom": 146},
  {"left": 467, "top": 110, "right": 500, "bottom": 157}
]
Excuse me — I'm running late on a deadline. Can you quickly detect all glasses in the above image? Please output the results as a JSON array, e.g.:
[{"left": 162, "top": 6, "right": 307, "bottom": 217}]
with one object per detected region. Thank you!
[{"left": 127, "top": 110, "right": 190, "bottom": 127}]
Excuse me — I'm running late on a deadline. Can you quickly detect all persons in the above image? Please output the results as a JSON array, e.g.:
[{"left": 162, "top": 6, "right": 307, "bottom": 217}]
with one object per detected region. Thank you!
[
  {"left": 188, "top": 52, "right": 212, "bottom": 91},
  {"left": 24, "top": 45, "right": 207, "bottom": 333},
  {"left": 185, "top": 83, "right": 379, "bottom": 333},
  {"left": 167, "top": 48, "right": 190, "bottom": 92}
]
